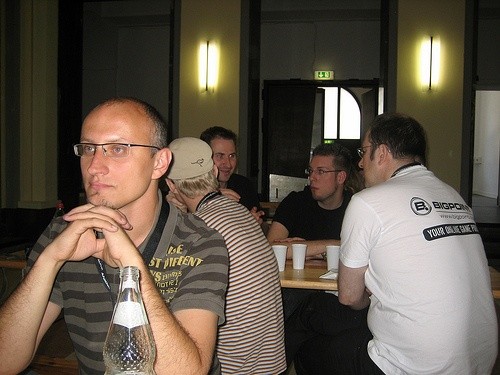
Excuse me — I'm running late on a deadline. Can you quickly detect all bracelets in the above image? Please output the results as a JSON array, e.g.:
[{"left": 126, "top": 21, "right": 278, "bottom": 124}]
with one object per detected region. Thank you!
[{"left": 320, "top": 253, "right": 327, "bottom": 259}]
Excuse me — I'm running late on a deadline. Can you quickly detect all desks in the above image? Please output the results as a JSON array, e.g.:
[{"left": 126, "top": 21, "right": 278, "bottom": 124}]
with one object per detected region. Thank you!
[{"left": 0, "top": 242, "right": 500, "bottom": 299}]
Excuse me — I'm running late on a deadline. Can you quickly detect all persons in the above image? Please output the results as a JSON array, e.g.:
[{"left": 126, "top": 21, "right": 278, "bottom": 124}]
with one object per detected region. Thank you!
[
  {"left": 0, "top": 97, "right": 228, "bottom": 375},
  {"left": 285, "top": 112, "right": 498, "bottom": 375},
  {"left": 200, "top": 125, "right": 266, "bottom": 227},
  {"left": 269, "top": 143, "right": 355, "bottom": 260},
  {"left": 164, "top": 136, "right": 287, "bottom": 375}
]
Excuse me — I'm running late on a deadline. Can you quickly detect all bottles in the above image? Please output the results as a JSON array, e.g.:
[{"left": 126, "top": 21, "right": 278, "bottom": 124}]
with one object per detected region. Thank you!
[{"left": 104, "top": 266, "right": 157, "bottom": 375}]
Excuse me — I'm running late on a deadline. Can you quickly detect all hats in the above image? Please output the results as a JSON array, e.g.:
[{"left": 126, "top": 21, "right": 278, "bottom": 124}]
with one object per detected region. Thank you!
[{"left": 166, "top": 137, "right": 214, "bottom": 178}]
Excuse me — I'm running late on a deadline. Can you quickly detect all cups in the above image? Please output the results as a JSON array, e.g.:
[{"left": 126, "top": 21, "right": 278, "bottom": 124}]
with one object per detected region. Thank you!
[
  {"left": 292, "top": 244, "right": 307, "bottom": 269},
  {"left": 272, "top": 245, "right": 287, "bottom": 271},
  {"left": 326, "top": 246, "right": 340, "bottom": 271}
]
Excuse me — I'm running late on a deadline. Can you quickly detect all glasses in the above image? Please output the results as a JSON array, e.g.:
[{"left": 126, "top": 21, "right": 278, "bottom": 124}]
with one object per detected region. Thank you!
[
  {"left": 305, "top": 168, "right": 342, "bottom": 176},
  {"left": 357, "top": 143, "right": 384, "bottom": 161},
  {"left": 73, "top": 141, "right": 161, "bottom": 158}
]
